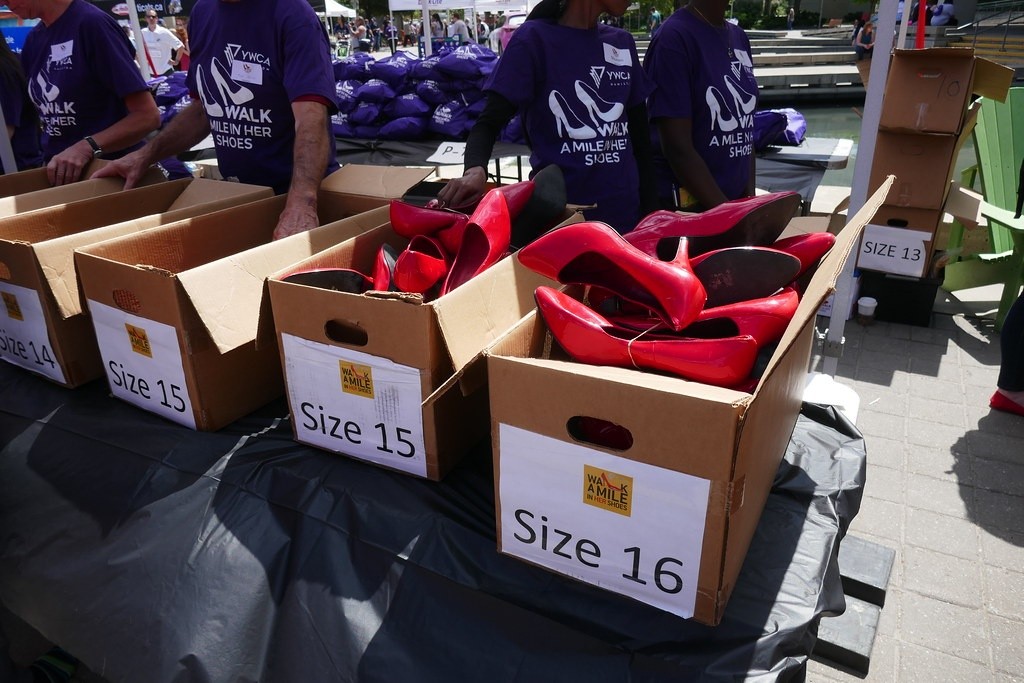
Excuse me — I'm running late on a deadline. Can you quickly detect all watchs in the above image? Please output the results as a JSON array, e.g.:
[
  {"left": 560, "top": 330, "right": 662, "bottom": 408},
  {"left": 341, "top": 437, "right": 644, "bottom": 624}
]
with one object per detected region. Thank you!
[{"left": 84, "top": 136, "right": 103, "bottom": 158}]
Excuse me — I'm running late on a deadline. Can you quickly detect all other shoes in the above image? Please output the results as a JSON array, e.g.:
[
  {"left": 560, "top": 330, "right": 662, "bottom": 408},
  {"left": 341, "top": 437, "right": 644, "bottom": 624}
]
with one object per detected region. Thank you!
[{"left": 990, "top": 391, "right": 1024, "bottom": 416}]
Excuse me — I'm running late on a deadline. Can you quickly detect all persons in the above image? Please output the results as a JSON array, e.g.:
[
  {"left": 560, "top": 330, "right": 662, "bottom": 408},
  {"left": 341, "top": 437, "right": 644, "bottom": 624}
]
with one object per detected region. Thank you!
[
  {"left": 850, "top": 0, "right": 959, "bottom": 62},
  {"left": 438, "top": 0, "right": 658, "bottom": 234},
  {"left": 650, "top": 7, "right": 661, "bottom": 36},
  {"left": 642, "top": 0, "right": 759, "bottom": 213},
  {"left": 0, "top": 27, "right": 26, "bottom": 175},
  {"left": 122, "top": 10, "right": 190, "bottom": 78},
  {"left": 0, "top": 0, "right": 161, "bottom": 186},
  {"left": 598, "top": 12, "right": 625, "bottom": 29},
  {"left": 990, "top": 290, "right": 1024, "bottom": 417},
  {"left": 788, "top": 5, "right": 795, "bottom": 30},
  {"left": 90, "top": 0, "right": 342, "bottom": 239},
  {"left": 333, "top": 9, "right": 514, "bottom": 55}
]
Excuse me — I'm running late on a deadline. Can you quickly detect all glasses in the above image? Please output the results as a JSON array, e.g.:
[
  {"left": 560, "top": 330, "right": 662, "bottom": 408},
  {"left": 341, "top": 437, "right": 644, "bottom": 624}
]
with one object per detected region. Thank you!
[{"left": 146, "top": 14, "right": 158, "bottom": 19}]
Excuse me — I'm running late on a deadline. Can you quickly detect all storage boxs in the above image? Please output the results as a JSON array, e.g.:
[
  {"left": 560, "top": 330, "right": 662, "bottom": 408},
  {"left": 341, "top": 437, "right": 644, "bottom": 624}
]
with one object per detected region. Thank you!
[
  {"left": 419, "top": 33, "right": 464, "bottom": 57},
  {"left": 1, "top": 159, "right": 898, "bottom": 627},
  {"left": 816, "top": 47, "right": 1016, "bottom": 326}
]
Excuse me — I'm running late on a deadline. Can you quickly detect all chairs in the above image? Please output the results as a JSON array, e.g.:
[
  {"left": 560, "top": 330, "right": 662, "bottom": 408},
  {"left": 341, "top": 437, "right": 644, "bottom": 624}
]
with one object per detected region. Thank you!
[{"left": 941, "top": 86, "right": 1024, "bottom": 330}]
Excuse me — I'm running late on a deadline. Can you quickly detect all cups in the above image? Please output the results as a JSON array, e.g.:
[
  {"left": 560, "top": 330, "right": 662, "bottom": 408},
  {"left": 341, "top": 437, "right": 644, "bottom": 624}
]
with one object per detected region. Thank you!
[{"left": 858, "top": 297, "right": 877, "bottom": 315}]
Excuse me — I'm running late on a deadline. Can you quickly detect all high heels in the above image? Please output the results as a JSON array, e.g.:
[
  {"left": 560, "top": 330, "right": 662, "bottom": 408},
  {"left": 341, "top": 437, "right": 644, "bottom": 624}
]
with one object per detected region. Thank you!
[
  {"left": 281, "top": 165, "right": 567, "bottom": 299},
  {"left": 517, "top": 191, "right": 836, "bottom": 394}
]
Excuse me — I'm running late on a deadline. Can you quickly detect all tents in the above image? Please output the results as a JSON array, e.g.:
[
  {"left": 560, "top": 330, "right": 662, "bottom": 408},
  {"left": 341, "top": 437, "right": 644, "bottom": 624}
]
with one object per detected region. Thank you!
[
  {"left": 389, "top": 0, "right": 542, "bottom": 58},
  {"left": 312, "top": 0, "right": 356, "bottom": 51}
]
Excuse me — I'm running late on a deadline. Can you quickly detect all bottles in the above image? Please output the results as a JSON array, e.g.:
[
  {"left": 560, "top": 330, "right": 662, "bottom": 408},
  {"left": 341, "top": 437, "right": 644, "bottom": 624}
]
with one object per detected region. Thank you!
[{"left": 336, "top": 40, "right": 350, "bottom": 58}]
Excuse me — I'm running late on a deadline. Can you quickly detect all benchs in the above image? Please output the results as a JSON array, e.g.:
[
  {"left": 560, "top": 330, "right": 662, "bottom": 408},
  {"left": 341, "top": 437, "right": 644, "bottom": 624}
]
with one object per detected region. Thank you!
[
  {"left": 828, "top": 18, "right": 843, "bottom": 27},
  {"left": 895, "top": 25, "right": 963, "bottom": 48}
]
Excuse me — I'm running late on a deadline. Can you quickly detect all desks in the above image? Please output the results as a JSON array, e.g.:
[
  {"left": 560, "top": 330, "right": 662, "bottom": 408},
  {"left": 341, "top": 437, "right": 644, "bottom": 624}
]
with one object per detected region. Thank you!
[
  {"left": 1, "top": 359, "right": 868, "bottom": 683},
  {"left": 756, "top": 136, "right": 854, "bottom": 214},
  {"left": 335, "top": 137, "right": 533, "bottom": 187}
]
